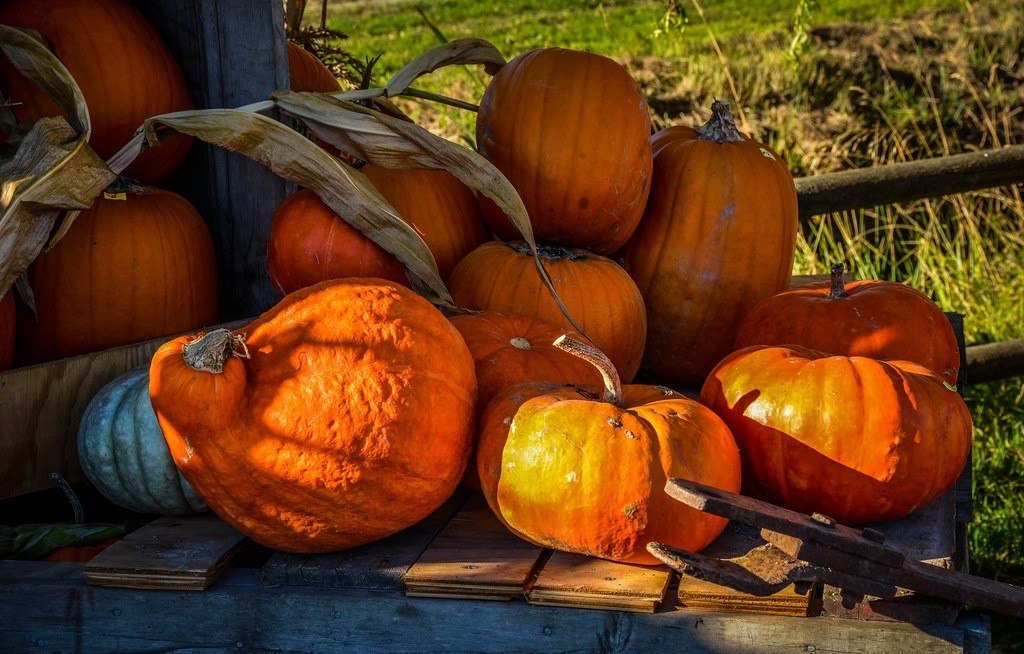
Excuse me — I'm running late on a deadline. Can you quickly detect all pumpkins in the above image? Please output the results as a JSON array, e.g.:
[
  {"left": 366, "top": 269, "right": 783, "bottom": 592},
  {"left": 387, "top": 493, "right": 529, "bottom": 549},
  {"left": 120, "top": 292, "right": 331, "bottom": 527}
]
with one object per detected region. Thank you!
[{"left": 0, "top": 0, "right": 972, "bottom": 565}]
